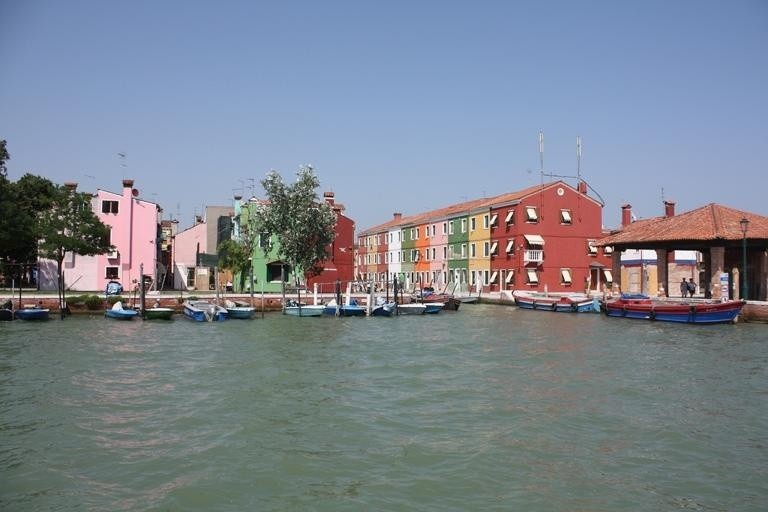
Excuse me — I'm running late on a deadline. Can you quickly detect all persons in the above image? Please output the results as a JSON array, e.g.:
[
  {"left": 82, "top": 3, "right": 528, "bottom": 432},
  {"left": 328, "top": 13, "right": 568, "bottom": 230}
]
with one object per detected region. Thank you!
[
  {"left": 688, "top": 278, "right": 697, "bottom": 297},
  {"left": 681, "top": 277, "right": 688, "bottom": 297}
]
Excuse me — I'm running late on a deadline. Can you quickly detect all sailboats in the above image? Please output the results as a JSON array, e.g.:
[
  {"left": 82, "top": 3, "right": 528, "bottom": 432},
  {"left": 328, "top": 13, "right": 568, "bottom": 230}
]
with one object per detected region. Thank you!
[{"left": 321, "top": 278, "right": 461, "bottom": 317}]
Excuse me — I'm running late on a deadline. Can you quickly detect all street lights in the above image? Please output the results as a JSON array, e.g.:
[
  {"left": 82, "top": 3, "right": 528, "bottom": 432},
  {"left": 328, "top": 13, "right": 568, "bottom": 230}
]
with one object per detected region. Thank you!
[{"left": 739, "top": 214, "right": 749, "bottom": 299}]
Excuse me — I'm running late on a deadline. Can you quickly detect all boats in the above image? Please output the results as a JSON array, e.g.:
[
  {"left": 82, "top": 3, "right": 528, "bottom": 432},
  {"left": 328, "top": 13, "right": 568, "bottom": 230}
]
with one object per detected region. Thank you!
[
  {"left": 510, "top": 287, "right": 594, "bottom": 315},
  {"left": 180, "top": 297, "right": 227, "bottom": 322},
  {"left": 143, "top": 305, "right": 175, "bottom": 320},
  {"left": 15, "top": 307, "right": 49, "bottom": 321},
  {"left": 0, "top": 308, "right": 15, "bottom": 321},
  {"left": 223, "top": 298, "right": 256, "bottom": 320},
  {"left": 280, "top": 299, "right": 326, "bottom": 317},
  {"left": 105, "top": 301, "right": 138, "bottom": 320},
  {"left": 596, "top": 294, "right": 747, "bottom": 325}
]
